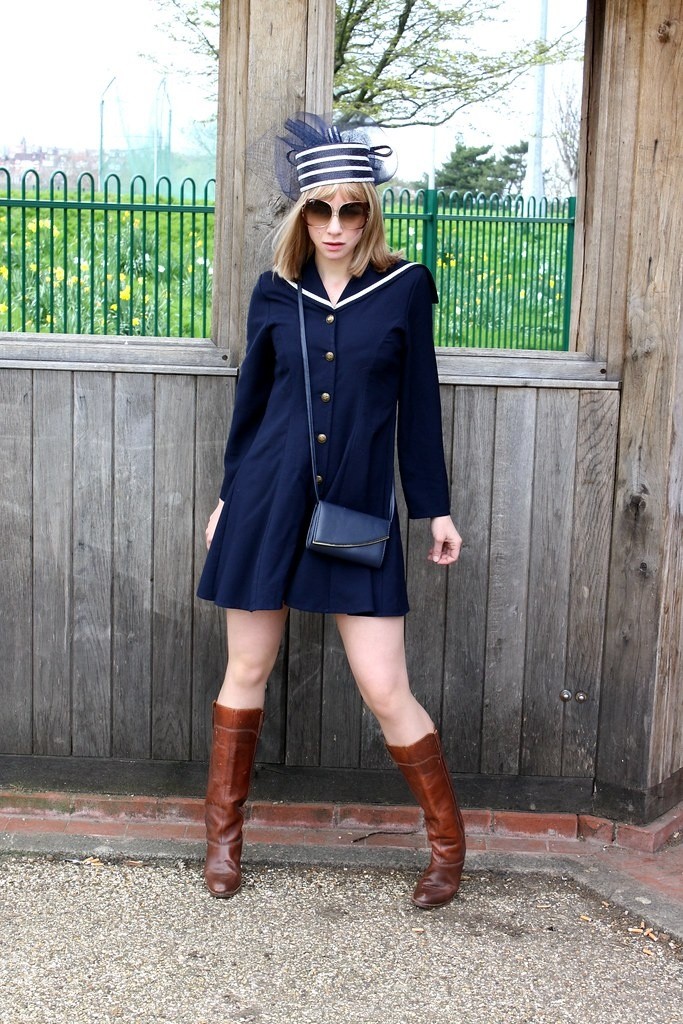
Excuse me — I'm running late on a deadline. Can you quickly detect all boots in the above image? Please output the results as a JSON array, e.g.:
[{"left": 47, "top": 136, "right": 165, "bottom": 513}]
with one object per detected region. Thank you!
[
  {"left": 385, "top": 728, "right": 466, "bottom": 910},
  {"left": 204, "top": 700, "right": 266, "bottom": 898}
]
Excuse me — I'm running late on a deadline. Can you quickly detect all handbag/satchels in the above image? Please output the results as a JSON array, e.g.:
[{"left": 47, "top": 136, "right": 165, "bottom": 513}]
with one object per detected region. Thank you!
[{"left": 306, "top": 501, "right": 392, "bottom": 567}]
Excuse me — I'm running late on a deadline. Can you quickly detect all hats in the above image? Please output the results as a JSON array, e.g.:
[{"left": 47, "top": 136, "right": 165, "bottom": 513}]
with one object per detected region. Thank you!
[{"left": 273, "top": 110, "right": 397, "bottom": 202}]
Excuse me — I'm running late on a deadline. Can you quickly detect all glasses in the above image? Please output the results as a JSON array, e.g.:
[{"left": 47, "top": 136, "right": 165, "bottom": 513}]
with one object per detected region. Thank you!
[{"left": 301, "top": 199, "right": 369, "bottom": 230}]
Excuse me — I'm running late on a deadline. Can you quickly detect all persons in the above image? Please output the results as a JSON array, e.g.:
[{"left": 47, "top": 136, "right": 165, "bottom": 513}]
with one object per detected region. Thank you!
[{"left": 196, "top": 112, "right": 467, "bottom": 909}]
